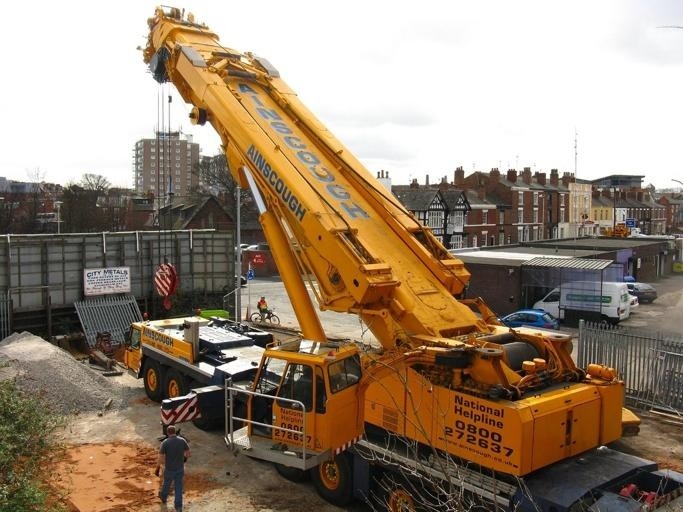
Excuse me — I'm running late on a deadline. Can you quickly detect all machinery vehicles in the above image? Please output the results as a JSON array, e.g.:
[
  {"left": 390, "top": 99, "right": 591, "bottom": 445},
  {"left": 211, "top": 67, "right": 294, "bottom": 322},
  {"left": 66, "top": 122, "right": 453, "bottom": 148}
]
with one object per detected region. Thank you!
[{"left": 123, "top": 4, "right": 682, "bottom": 512}]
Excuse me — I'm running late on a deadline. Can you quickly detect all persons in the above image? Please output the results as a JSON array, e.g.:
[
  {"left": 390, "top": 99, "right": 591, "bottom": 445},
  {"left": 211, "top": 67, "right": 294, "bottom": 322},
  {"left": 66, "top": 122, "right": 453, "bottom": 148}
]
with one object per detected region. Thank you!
[
  {"left": 258, "top": 296, "right": 272, "bottom": 322},
  {"left": 155, "top": 425, "right": 190, "bottom": 512}
]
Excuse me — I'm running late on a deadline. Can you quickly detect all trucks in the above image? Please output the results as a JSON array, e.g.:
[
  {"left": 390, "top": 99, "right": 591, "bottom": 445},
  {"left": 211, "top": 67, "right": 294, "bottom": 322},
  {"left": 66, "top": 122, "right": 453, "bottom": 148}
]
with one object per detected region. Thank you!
[{"left": 532, "top": 280, "right": 629, "bottom": 329}]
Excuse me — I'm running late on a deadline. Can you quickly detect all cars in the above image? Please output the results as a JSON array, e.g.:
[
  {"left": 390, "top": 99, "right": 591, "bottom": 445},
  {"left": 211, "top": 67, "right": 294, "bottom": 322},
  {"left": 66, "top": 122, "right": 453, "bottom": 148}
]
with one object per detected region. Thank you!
[
  {"left": 626, "top": 283, "right": 657, "bottom": 302},
  {"left": 628, "top": 293, "right": 639, "bottom": 309},
  {"left": 498, "top": 309, "right": 559, "bottom": 330}
]
